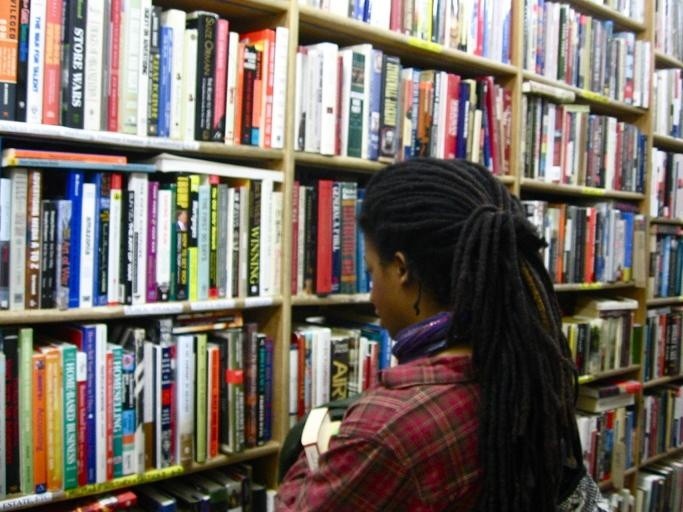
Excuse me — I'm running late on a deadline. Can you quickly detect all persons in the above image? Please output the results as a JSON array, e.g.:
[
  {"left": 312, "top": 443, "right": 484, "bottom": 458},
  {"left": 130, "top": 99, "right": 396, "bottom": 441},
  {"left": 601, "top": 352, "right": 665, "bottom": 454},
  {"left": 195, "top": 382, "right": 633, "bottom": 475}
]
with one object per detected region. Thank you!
[{"left": 276, "top": 156, "right": 602, "bottom": 512}]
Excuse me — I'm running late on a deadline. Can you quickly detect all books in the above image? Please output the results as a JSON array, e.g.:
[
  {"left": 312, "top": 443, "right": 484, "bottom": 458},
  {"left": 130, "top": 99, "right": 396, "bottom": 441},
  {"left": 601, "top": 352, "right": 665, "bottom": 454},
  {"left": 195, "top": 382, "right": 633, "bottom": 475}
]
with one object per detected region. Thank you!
[
  {"left": 0, "top": 1, "right": 289, "bottom": 511},
  {"left": 290, "top": 0, "right": 514, "bottom": 466},
  {"left": 525, "top": 0, "right": 683, "bottom": 512}
]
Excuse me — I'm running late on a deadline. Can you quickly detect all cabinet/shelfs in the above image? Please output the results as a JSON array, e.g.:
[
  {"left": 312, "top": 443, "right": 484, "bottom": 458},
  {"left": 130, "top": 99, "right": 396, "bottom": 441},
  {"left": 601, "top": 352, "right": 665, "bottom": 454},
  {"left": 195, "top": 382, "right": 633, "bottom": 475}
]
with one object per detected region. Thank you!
[{"left": 0, "top": 0, "right": 683, "bottom": 511}]
